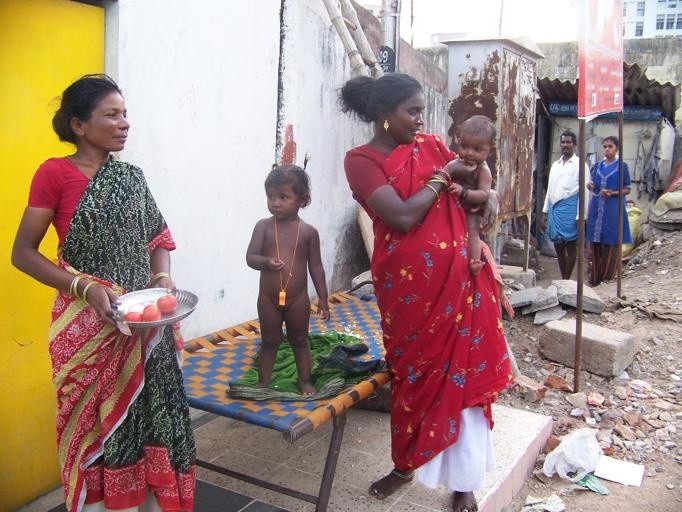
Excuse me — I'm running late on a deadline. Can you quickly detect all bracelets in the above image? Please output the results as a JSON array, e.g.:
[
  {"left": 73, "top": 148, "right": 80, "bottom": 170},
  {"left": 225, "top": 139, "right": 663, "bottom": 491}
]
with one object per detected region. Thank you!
[
  {"left": 148, "top": 271, "right": 173, "bottom": 286},
  {"left": 66, "top": 275, "right": 96, "bottom": 300}
]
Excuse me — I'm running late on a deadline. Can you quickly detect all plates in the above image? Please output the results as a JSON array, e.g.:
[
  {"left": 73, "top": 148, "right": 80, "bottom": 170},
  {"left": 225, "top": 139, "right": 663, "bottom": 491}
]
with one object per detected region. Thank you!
[{"left": 110, "top": 286, "right": 200, "bottom": 331}]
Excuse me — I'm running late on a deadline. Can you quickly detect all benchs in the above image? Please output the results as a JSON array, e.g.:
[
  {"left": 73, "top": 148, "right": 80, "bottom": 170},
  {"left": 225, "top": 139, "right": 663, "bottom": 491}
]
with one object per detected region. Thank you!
[{"left": 175, "top": 282, "right": 401, "bottom": 510}]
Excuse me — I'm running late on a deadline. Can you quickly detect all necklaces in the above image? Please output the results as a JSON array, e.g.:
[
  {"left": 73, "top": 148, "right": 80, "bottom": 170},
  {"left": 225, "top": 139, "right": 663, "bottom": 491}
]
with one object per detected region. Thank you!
[{"left": 272, "top": 216, "right": 302, "bottom": 307}]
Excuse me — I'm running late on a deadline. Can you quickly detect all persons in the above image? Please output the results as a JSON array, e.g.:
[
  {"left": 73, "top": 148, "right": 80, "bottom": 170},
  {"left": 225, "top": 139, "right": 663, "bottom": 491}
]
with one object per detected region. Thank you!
[
  {"left": 542, "top": 130, "right": 633, "bottom": 287},
  {"left": 10, "top": 76, "right": 197, "bottom": 512},
  {"left": 246, "top": 154, "right": 329, "bottom": 395}
]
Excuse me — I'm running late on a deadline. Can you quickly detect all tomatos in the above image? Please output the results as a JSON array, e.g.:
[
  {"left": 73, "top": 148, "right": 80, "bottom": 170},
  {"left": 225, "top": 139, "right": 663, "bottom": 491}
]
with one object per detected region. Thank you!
[{"left": 122, "top": 294, "right": 178, "bottom": 323}]
